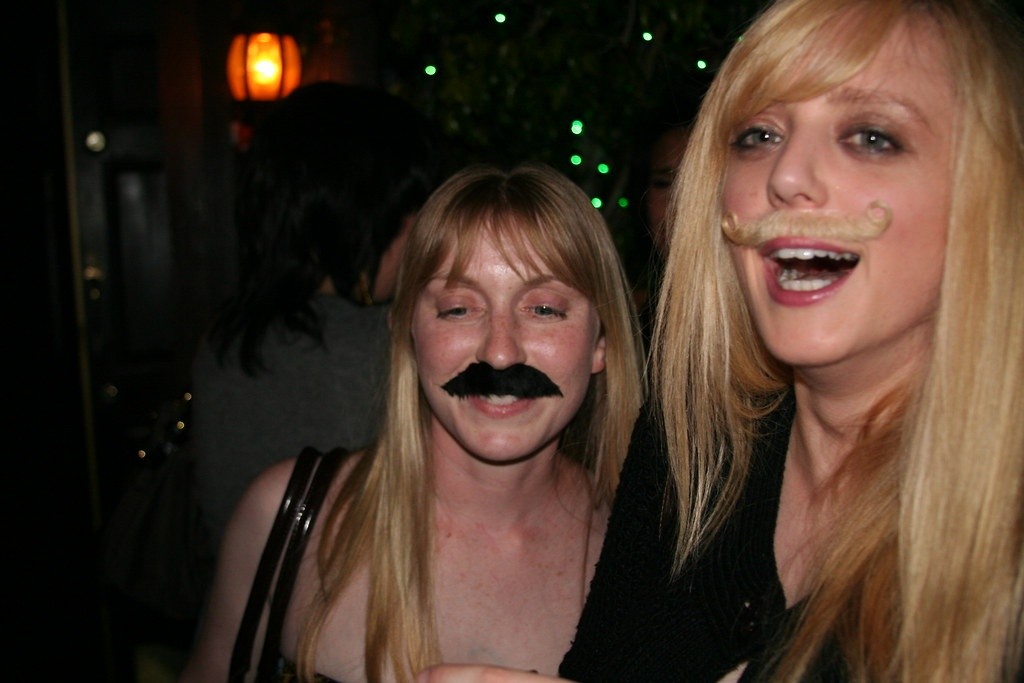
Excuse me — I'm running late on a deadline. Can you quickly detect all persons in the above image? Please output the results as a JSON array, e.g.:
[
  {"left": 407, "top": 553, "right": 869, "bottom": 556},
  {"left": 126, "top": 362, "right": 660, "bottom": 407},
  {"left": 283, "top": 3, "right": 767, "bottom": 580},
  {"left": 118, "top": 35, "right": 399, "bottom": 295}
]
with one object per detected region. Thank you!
[
  {"left": 417, "top": 0, "right": 1024, "bottom": 683},
  {"left": 188, "top": 79, "right": 688, "bottom": 683}
]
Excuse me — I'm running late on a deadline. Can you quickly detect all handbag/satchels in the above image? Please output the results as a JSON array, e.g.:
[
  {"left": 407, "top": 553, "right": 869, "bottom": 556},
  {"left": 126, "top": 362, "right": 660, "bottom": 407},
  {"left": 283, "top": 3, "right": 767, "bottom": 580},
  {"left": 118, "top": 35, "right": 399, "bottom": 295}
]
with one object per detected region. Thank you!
[{"left": 96, "top": 442, "right": 210, "bottom": 639}]
